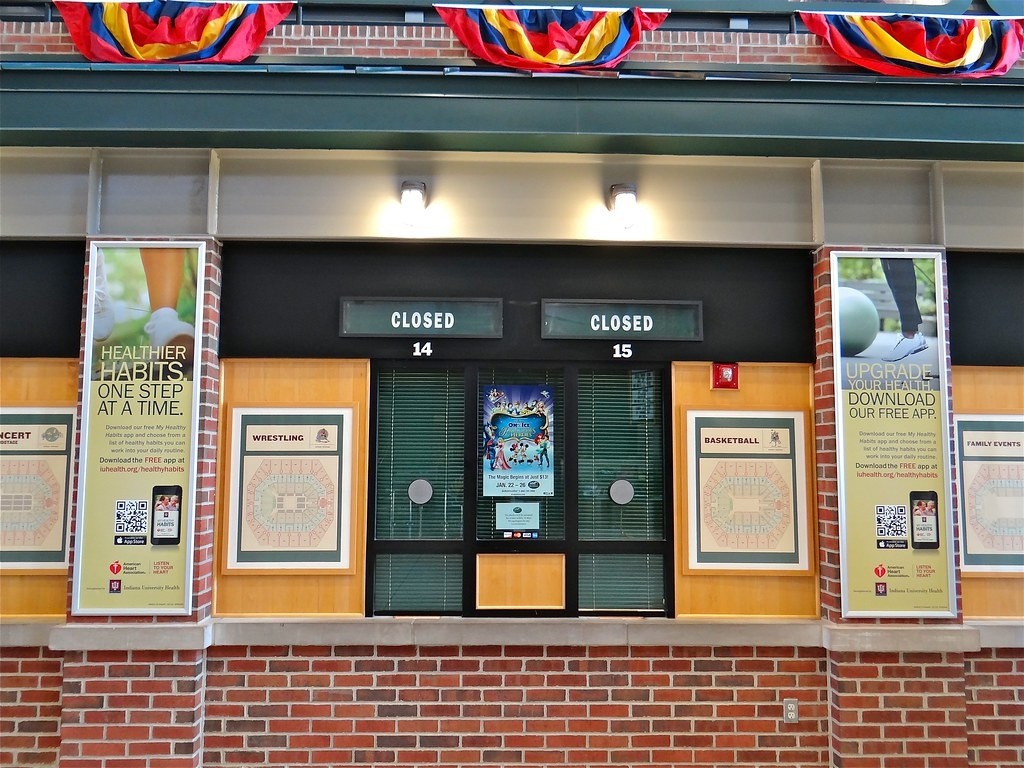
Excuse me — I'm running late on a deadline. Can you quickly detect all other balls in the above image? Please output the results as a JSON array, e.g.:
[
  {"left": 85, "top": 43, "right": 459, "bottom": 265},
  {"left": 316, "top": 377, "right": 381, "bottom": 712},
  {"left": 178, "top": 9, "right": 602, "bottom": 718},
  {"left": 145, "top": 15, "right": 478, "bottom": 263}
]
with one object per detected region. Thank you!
[{"left": 838, "top": 286, "right": 880, "bottom": 357}]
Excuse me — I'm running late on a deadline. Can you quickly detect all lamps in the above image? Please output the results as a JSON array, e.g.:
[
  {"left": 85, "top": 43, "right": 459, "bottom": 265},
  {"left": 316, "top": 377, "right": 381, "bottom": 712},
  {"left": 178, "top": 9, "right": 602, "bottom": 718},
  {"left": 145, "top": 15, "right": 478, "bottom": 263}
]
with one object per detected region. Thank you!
[
  {"left": 400, "top": 179, "right": 428, "bottom": 209},
  {"left": 608, "top": 182, "right": 638, "bottom": 212}
]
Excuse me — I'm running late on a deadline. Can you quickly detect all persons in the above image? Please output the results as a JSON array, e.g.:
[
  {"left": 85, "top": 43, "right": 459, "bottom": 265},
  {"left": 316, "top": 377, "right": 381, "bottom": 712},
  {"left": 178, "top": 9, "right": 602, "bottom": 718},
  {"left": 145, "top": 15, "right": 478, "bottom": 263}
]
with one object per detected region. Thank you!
[
  {"left": 880, "top": 258, "right": 929, "bottom": 362},
  {"left": 94, "top": 249, "right": 195, "bottom": 364}
]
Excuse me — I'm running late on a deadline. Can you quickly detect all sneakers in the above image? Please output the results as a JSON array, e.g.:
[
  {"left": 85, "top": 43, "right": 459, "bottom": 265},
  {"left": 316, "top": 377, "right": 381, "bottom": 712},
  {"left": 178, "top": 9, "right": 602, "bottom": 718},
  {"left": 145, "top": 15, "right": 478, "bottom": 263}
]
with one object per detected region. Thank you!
[
  {"left": 93, "top": 248, "right": 114, "bottom": 342},
  {"left": 144, "top": 311, "right": 195, "bottom": 377},
  {"left": 881, "top": 332, "right": 929, "bottom": 362}
]
